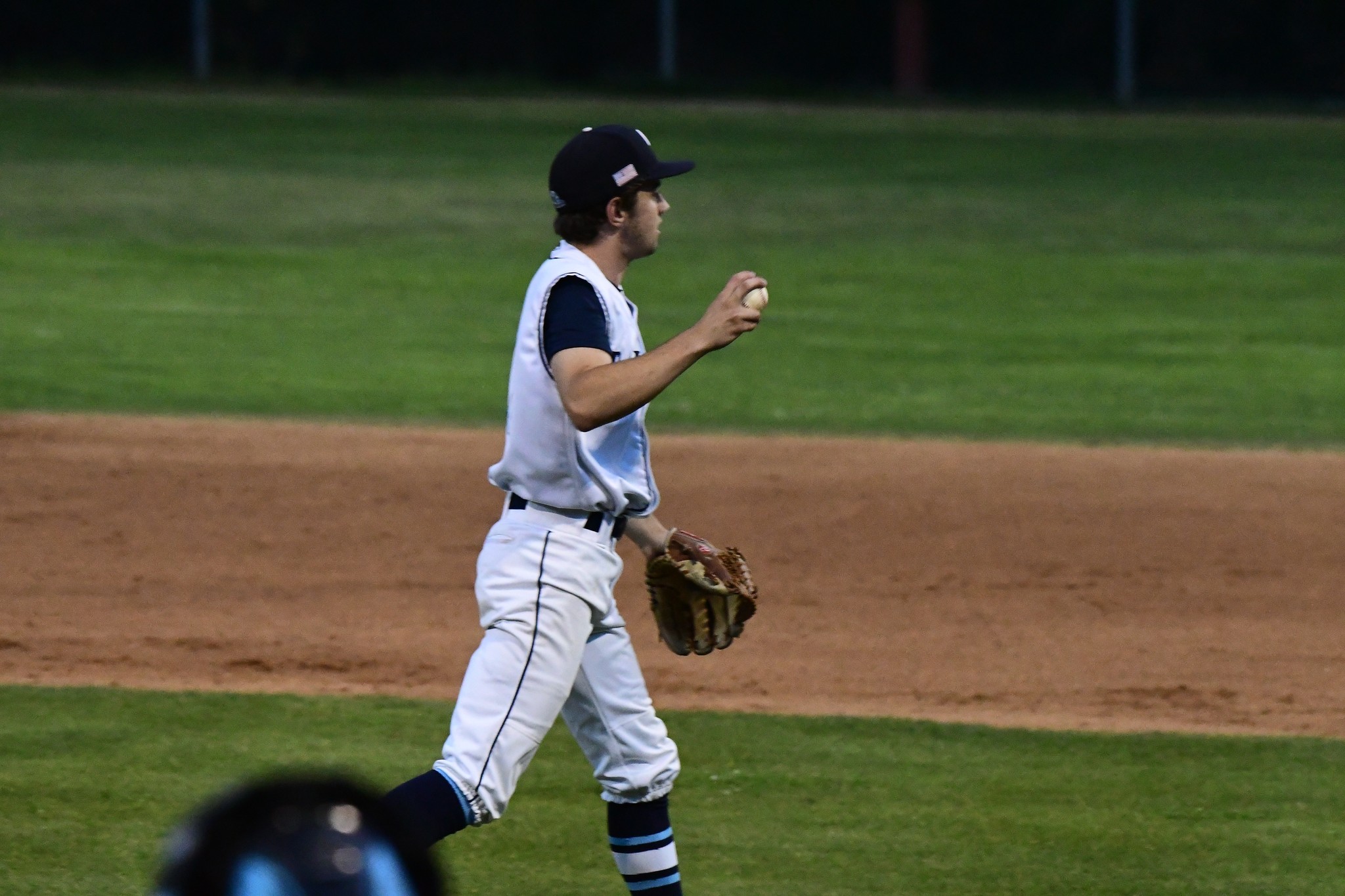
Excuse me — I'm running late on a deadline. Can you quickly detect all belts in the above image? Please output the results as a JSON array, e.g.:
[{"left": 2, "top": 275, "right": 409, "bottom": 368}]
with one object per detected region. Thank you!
[{"left": 508, "top": 494, "right": 628, "bottom": 542}]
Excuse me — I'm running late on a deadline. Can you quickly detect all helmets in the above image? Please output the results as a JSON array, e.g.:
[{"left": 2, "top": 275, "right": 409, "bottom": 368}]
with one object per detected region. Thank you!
[{"left": 156, "top": 774, "right": 442, "bottom": 896}]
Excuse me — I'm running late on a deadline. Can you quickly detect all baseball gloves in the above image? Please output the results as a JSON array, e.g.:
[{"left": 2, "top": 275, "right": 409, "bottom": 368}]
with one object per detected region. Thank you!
[{"left": 644, "top": 528, "right": 758, "bottom": 658}]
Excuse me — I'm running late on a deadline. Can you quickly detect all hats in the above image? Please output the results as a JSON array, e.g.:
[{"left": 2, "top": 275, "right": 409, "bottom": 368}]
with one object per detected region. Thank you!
[{"left": 550, "top": 121, "right": 695, "bottom": 211}]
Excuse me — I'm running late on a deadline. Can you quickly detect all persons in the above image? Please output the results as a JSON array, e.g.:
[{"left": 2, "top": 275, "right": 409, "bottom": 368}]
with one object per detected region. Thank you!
[
  {"left": 383, "top": 122, "right": 767, "bottom": 896},
  {"left": 132, "top": 764, "right": 449, "bottom": 896}
]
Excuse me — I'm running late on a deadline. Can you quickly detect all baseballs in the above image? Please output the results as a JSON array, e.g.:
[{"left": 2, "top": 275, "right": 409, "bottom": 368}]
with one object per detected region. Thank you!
[{"left": 745, "top": 289, "right": 770, "bottom": 311}]
